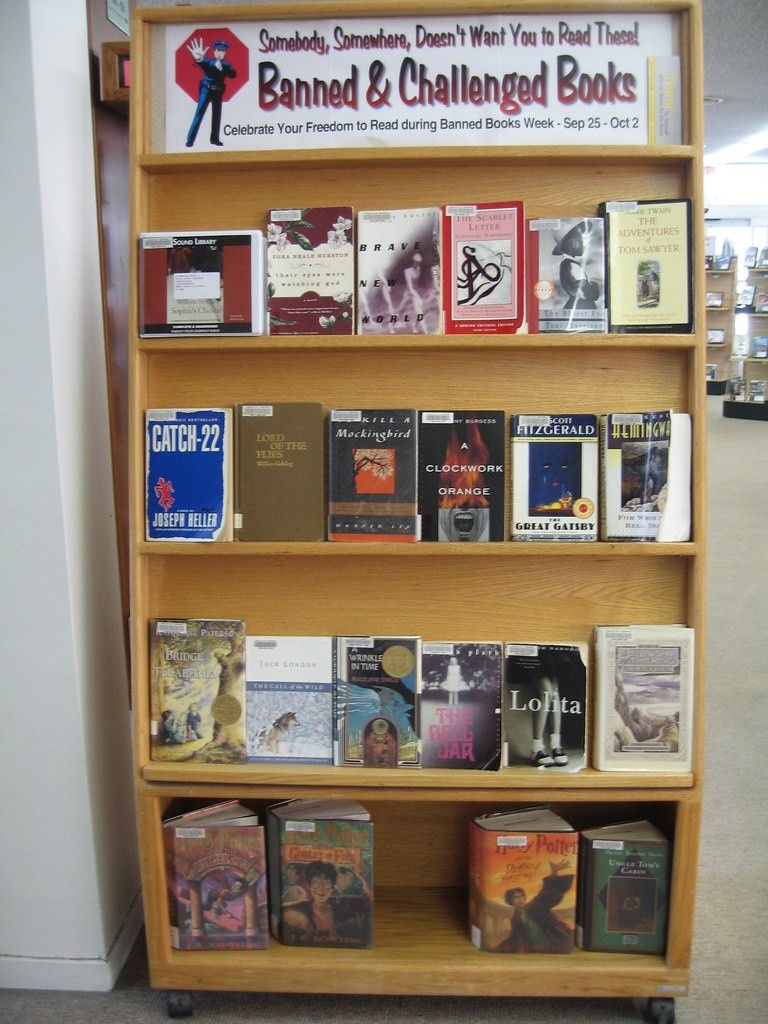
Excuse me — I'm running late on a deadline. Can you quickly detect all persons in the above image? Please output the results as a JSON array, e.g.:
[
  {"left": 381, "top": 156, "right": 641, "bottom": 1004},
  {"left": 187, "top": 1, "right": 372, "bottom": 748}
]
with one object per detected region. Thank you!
[
  {"left": 641, "top": 273, "right": 659, "bottom": 302},
  {"left": 552, "top": 220, "right": 600, "bottom": 310}
]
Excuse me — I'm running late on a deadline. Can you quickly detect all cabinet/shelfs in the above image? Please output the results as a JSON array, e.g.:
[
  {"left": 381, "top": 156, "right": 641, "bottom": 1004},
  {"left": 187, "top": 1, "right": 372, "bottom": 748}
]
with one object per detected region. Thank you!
[{"left": 129, "top": 0, "right": 768, "bottom": 1024}]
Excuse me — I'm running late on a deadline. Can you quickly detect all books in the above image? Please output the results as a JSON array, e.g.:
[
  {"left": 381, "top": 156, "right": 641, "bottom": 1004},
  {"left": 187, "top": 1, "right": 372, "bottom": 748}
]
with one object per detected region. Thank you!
[
  {"left": 139, "top": 615, "right": 700, "bottom": 777},
  {"left": 131, "top": 387, "right": 696, "bottom": 548},
  {"left": 128, "top": 196, "right": 696, "bottom": 344},
  {"left": 703, "top": 234, "right": 768, "bottom": 404},
  {"left": 148, "top": 797, "right": 673, "bottom": 963}
]
